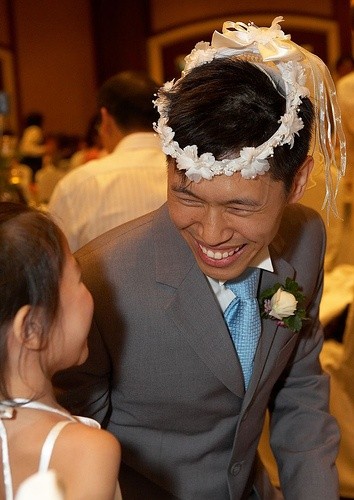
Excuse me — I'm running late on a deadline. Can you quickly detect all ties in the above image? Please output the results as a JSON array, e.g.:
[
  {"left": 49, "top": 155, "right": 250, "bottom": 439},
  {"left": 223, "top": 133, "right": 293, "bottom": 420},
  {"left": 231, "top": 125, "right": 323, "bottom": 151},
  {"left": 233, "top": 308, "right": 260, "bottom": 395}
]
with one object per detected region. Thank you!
[{"left": 223, "top": 267, "right": 261, "bottom": 391}]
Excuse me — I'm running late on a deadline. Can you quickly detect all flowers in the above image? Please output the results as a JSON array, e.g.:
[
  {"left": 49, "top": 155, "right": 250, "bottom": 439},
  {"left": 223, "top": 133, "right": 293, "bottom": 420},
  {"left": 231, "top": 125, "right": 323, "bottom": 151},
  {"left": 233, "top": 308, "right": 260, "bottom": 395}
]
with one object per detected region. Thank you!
[
  {"left": 153, "top": 16, "right": 310, "bottom": 183},
  {"left": 260, "top": 277, "right": 312, "bottom": 333}
]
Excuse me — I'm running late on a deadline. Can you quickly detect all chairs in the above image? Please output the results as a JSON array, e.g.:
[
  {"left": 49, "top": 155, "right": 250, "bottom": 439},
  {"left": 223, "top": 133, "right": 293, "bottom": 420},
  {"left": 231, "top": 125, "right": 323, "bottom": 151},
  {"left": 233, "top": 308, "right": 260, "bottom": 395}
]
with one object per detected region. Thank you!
[
  {"left": 295, "top": 163, "right": 347, "bottom": 274},
  {"left": 9, "top": 149, "right": 99, "bottom": 216}
]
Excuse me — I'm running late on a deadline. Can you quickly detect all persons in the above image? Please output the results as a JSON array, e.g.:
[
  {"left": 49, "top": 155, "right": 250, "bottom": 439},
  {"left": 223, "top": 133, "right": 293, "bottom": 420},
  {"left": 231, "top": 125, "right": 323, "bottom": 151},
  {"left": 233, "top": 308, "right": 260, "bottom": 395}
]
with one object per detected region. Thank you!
[
  {"left": 0, "top": 200, "right": 122, "bottom": 500},
  {"left": 51, "top": 16, "right": 347, "bottom": 500},
  {"left": 0, "top": 50, "right": 354, "bottom": 256}
]
